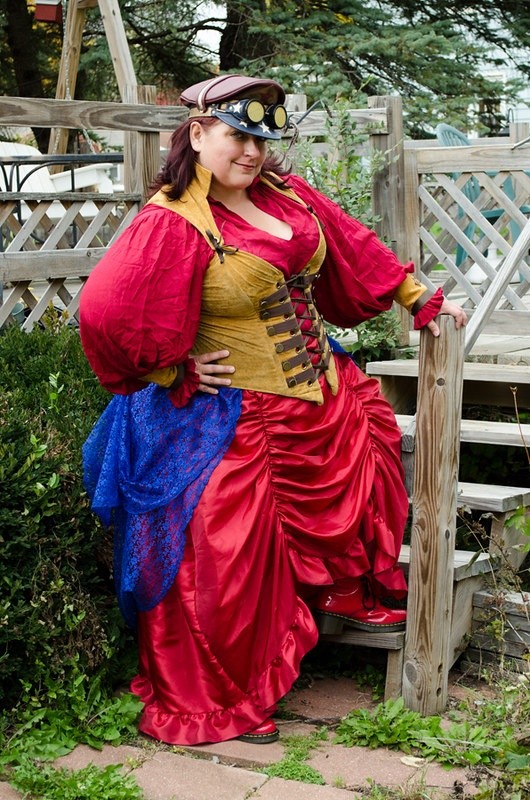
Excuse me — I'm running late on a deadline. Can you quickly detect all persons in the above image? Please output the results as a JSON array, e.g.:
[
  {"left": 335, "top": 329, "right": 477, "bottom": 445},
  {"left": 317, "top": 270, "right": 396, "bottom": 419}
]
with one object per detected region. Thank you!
[{"left": 76, "top": 71, "right": 471, "bottom": 752}]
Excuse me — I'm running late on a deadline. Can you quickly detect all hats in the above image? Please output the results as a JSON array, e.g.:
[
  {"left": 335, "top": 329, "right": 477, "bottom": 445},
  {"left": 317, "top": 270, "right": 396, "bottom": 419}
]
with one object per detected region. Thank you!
[{"left": 180, "top": 75, "right": 289, "bottom": 140}]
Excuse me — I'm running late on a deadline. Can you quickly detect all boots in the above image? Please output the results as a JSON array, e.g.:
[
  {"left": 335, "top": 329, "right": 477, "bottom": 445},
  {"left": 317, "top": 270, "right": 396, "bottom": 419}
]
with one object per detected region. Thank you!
[
  {"left": 316, "top": 576, "right": 408, "bottom": 636},
  {"left": 236, "top": 718, "right": 279, "bottom": 743}
]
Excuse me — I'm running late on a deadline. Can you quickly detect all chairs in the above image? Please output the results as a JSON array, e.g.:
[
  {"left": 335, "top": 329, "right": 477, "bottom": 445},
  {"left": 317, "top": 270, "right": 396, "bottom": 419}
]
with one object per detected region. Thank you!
[
  {"left": 436, "top": 120, "right": 528, "bottom": 286},
  {"left": 0, "top": 141, "right": 117, "bottom": 224}
]
